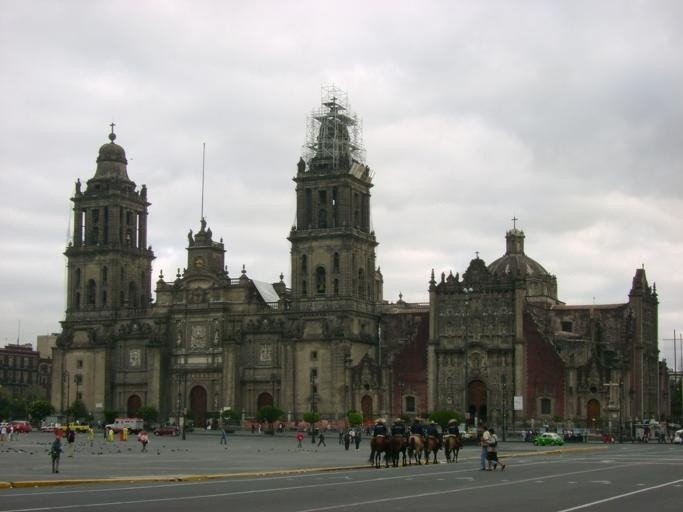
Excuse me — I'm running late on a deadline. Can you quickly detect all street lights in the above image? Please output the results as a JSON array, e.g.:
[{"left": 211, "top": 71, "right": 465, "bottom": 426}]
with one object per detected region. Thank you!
[
  {"left": 63, "top": 370, "right": 69, "bottom": 421},
  {"left": 619, "top": 374, "right": 624, "bottom": 442},
  {"left": 310, "top": 368, "right": 315, "bottom": 443}
]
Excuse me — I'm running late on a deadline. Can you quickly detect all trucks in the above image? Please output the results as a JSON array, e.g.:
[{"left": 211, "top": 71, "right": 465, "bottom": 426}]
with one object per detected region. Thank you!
[{"left": 104, "top": 417, "right": 145, "bottom": 434}]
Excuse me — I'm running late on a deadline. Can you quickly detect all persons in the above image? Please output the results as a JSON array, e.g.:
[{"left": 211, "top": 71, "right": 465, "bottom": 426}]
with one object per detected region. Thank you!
[
  {"left": 481, "top": 427, "right": 506, "bottom": 470},
  {"left": 218, "top": 427, "right": 226, "bottom": 444},
  {"left": 137, "top": 430, "right": 149, "bottom": 453},
  {"left": 13, "top": 424, "right": 21, "bottom": 440},
  {"left": 0, "top": 422, "right": 12, "bottom": 441},
  {"left": 317, "top": 431, "right": 326, "bottom": 446},
  {"left": 478, "top": 426, "right": 497, "bottom": 471},
  {"left": 296, "top": 429, "right": 303, "bottom": 448},
  {"left": 50, "top": 435, "right": 64, "bottom": 474},
  {"left": 61, "top": 423, "right": 129, "bottom": 457},
  {"left": 337, "top": 417, "right": 463, "bottom": 449}
]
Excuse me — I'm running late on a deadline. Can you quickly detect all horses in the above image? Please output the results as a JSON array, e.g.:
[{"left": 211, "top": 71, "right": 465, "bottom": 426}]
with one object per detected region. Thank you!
[{"left": 368, "top": 434, "right": 460, "bottom": 469}]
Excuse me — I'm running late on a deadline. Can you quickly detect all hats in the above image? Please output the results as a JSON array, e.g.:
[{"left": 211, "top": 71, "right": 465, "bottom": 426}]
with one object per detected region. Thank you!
[
  {"left": 393, "top": 417, "right": 405, "bottom": 424},
  {"left": 429, "top": 420, "right": 438, "bottom": 425},
  {"left": 375, "top": 418, "right": 385, "bottom": 424},
  {"left": 447, "top": 417, "right": 459, "bottom": 424},
  {"left": 415, "top": 415, "right": 425, "bottom": 422}
]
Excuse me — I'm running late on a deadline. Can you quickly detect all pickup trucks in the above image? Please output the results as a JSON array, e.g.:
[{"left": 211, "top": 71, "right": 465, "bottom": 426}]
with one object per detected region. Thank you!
[{"left": 60, "top": 422, "right": 89, "bottom": 433}]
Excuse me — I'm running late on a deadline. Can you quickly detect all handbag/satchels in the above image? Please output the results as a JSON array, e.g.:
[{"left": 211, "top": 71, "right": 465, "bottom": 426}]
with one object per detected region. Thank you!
[{"left": 488, "top": 440, "right": 498, "bottom": 448}]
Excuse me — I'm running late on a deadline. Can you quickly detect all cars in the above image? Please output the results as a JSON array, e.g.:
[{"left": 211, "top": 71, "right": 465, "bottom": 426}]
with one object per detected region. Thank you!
[
  {"left": 533, "top": 432, "right": 564, "bottom": 446},
  {"left": 6, "top": 421, "right": 32, "bottom": 433},
  {"left": 154, "top": 425, "right": 179, "bottom": 436}
]
